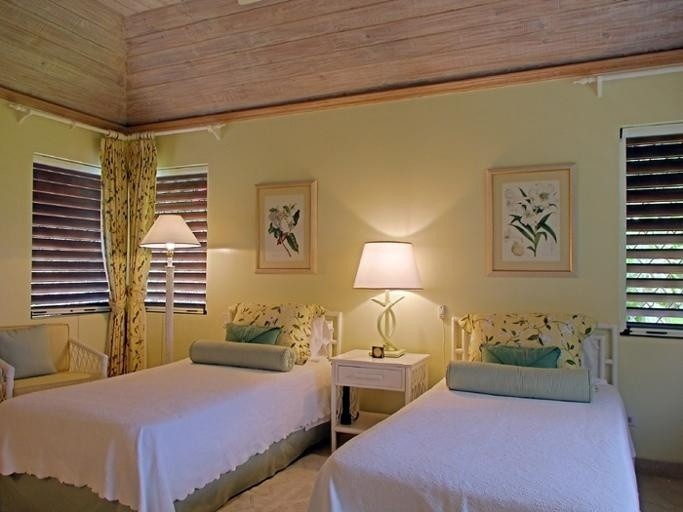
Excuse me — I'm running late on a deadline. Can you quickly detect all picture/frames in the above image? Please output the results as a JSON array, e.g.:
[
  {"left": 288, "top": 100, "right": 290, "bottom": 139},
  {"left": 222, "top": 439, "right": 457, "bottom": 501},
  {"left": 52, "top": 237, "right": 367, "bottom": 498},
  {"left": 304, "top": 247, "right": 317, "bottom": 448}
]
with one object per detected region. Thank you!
[
  {"left": 483, "top": 162, "right": 579, "bottom": 279},
  {"left": 253, "top": 180, "right": 320, "bottom": 275}
]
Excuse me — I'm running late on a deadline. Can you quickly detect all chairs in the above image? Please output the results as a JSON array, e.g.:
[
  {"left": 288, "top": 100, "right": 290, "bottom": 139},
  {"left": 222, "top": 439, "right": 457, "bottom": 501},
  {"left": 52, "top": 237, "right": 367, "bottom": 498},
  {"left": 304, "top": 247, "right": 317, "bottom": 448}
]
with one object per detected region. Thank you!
[{"left": 0, "top": 324, "right": 109, "bottom": 403}]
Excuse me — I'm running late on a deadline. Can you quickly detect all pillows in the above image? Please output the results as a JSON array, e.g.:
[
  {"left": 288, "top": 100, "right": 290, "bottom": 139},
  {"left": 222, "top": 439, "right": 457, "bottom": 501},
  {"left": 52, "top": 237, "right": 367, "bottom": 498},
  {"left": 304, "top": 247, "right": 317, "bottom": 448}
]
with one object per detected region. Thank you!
[
  {"left": 0, "top": 326, "right": 58, "bottom": 380},
  {"left": 482, "top": 345, "right": 561, "bottom": 368},
  {"left": 446, "top": 360, "right": 591, "bottom": 403},
  {"left": 225, "top": 323, "right": 282, "bottom": 345},
  {"left": 189, "top": 338, "right": 296, "bottom": 373},
  {"left": 456, "top": 311, "right": 596, "bottom": 369},
  {"left": 228, "top": 300, "right": 329, "bottom": 365}
]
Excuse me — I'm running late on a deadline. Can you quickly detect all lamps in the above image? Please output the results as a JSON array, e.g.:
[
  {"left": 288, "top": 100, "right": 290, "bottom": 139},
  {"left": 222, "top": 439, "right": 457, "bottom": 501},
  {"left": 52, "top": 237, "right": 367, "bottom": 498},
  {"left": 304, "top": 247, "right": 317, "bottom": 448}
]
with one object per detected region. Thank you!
[
  {"left": 350, "top": 241, "right": 423, "bottom": 358},
  {"left": 137, "top": 214, "right": 201, "bottom": 363}
]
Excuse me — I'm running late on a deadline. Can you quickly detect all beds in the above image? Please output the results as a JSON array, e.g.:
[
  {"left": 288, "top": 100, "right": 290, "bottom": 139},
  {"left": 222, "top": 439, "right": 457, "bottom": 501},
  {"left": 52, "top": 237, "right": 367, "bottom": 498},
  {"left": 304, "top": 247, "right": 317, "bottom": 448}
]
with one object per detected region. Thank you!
[
  {"left": 308, "top": 314, "right": 642, "bottom": 512},
  {"left": 0, "top": 305, "right": 343, "bottom": 512}
]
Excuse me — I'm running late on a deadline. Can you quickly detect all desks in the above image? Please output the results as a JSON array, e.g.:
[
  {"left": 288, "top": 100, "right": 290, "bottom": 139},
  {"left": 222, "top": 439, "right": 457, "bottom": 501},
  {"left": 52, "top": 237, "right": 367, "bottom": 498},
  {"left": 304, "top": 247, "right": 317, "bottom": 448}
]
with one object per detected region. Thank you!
[{"left": 329, "top": 349, "right": 431, "bottom": 453}]
